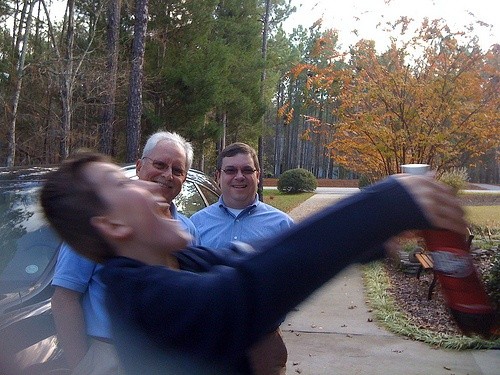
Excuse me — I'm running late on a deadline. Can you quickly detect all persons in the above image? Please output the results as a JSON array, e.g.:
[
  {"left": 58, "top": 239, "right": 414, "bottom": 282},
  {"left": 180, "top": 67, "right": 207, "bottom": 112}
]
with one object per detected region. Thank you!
[
  {"left": 50, "top": 131, "right": 294, "bottom": 375},
  {"left": 37, "top": 150, "right": 469, "bottom": 374}
]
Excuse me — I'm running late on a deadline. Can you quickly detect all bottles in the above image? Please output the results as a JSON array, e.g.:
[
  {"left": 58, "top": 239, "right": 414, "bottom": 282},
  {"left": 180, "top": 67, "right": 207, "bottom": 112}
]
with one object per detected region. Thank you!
[{"left": 400, "top": 163, "right": 496, "bottom": 340}]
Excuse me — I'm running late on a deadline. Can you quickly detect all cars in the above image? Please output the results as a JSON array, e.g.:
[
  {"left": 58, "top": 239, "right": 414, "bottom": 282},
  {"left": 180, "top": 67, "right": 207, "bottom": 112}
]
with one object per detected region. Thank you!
[{"left": 0, "top": 161, "right": 222, "bottom": 375}]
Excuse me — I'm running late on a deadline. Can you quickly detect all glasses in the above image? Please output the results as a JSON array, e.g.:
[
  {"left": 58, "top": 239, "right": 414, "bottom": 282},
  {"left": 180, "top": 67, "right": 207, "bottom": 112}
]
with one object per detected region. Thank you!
[
  {"left": 142, "top": 157, "right": 187, "bottom": 177},
  {"left": 221, "top": 166, "right": 256, "bottom": 175}
]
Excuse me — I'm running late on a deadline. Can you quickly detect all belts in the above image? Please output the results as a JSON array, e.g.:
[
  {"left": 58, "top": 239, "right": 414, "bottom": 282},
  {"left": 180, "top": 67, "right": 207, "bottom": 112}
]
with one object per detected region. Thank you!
[{"left": 87, "top": 335, "right": 115, "bottom": 346}]
